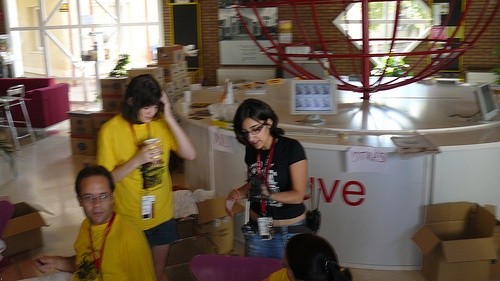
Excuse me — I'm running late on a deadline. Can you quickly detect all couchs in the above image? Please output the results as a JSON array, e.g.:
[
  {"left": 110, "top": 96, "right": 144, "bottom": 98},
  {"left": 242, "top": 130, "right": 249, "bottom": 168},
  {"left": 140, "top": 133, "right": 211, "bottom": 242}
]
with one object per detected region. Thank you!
[{"left": 0, "top": 77, "right": 70, "bottom": 129}]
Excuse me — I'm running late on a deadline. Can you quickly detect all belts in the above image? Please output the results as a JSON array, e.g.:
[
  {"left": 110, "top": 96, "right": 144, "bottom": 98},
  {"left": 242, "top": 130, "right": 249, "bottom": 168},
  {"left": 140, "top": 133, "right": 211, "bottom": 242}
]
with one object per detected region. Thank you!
[{"left": 248, "top": 216, "right": 307, "bottom": 235}]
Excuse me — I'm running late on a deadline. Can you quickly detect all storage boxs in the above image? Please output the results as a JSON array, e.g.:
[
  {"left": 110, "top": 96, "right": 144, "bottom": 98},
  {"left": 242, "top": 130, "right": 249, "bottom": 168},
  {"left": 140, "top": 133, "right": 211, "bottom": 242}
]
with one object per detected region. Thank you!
[
  {"left": 67, "top": 45, "right": 192, "bottom": 155},
  {"left": 180, "top": 195, "right": 246, "bottom": 254},
  {"left": 411, "top": 201, "right": 500, "bottom": 281},
  {"left": 1, "top": 201, "right": 55, "bottom": 258}
]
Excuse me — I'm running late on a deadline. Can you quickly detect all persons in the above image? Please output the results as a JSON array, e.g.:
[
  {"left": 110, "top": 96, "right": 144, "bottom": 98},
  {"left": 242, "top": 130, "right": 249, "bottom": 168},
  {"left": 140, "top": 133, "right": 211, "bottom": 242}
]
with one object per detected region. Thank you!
[
  {"left": 263, "top": 234, "right": 354, "bottom": 281},
  {"left": 34, "top": 165, "right": 158, "bottom": 281},
  {"left": 97, "top": 75, "right": 197, "bottom": 281},
  {"left": 224, "top": 99, "right": 309, "bottom": 260}
]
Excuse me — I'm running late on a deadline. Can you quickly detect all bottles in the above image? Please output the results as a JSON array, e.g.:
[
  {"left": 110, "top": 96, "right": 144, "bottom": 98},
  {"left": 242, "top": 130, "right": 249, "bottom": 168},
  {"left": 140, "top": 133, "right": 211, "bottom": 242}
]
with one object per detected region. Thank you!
[{"left": 224, "top": 79, "right": 235, "bottom": 104}]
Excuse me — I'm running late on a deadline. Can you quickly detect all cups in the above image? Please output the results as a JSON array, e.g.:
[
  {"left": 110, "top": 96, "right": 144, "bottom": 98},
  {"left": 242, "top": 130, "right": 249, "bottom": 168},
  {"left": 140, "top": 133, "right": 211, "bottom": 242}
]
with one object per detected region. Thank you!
[
  {"left": 143, "top": 139, "right": 163, "bottom": 159},
  {"left": 180, "top": 103, "right": 188, "bottom": 113},
  {"left": 183, "top": 91, "right": 192, "bottom": 102},
  {"left": 190, "top": 84, "right": 201, "bottom": 91}
]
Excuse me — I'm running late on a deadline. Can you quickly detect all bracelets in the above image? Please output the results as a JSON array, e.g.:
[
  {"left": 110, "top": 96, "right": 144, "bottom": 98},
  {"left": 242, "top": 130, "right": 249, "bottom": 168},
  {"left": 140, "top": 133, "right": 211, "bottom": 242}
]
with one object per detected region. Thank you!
[{"left": 233, "top": 189, "right": 240, "bottom": 201}]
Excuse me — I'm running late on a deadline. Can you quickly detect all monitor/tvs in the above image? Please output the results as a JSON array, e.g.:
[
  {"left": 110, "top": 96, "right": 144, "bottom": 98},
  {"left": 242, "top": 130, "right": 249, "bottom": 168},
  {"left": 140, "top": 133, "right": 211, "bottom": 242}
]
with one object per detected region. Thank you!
[
  {"left": 472, "top": 82, "right": 499, "bottom": 124},
  {"left": 290, "top": 80, "right": 337, "bottom": 126}
]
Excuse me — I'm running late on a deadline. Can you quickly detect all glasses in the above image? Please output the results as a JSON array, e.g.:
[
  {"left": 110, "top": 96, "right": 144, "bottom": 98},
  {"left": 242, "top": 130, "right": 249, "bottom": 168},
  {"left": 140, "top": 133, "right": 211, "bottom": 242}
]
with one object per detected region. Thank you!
[
  {"left": 83, "top": 195, "right": 111, "bottom": 203},
  {"left": 240, "top": 119, "right": 266, "bottom": 137}
]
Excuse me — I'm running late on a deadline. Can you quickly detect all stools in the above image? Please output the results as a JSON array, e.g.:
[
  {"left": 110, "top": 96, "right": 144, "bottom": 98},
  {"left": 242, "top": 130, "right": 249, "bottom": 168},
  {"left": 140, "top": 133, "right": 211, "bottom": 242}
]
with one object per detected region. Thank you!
[{"left": 0, "top": 84, "right": 36, "bottom": 151}]
